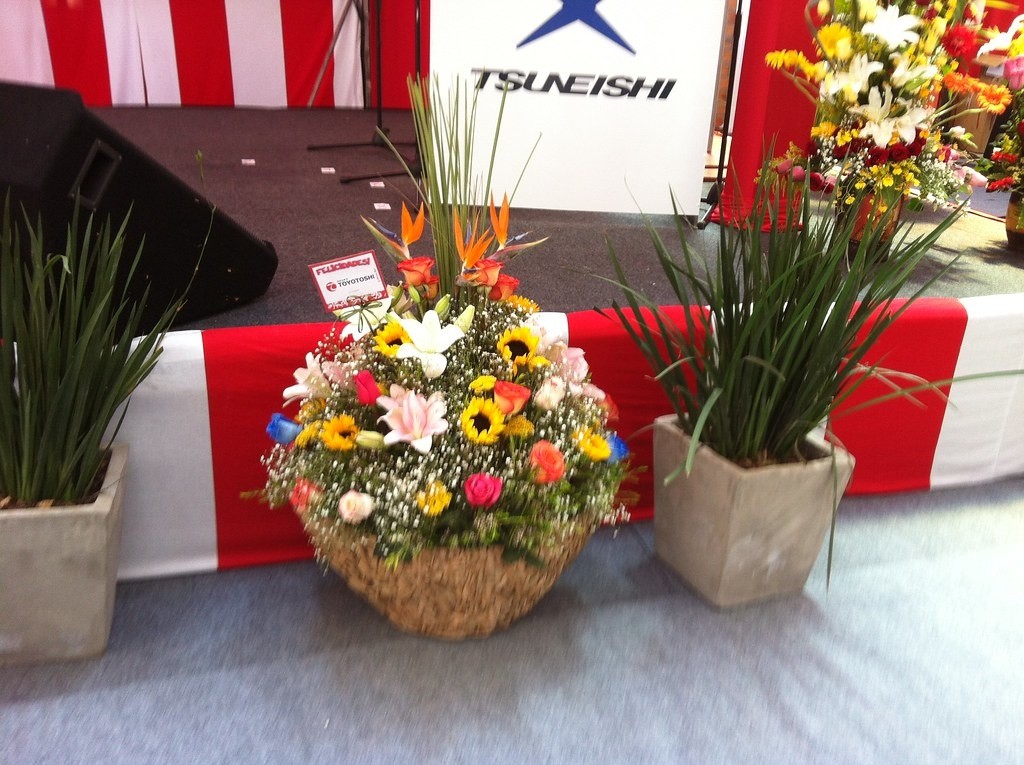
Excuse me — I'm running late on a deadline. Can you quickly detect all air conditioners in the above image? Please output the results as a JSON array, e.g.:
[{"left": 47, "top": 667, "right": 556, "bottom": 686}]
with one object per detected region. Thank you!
[{"left": 1, "top": 186, "right": 198, "bottom": 668}]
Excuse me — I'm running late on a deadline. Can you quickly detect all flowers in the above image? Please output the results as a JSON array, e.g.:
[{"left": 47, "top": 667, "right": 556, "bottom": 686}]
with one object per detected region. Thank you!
[
  {"left": 977, "top": 18, "right": 1023, "bottom": 193},
  {"left": 244, "top": 75, "right": 652, "bottom": 574},
  {"left": 755, "top": 0, "right": 998, "bottom": 222}
]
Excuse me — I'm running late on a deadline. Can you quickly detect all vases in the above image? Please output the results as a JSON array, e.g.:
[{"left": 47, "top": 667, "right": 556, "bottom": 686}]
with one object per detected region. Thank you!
[
  {"left": 1002, "top": 191, "right": 1024, "bottom": 254},
  {"left": 294, "top": 496, "right": 606, "bottom": 638},
  {"left": 831, "top": 180, "right": 903, "bottom": 262}
]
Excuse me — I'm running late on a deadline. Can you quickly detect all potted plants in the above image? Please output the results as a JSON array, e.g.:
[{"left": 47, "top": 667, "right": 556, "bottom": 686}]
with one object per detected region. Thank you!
[{"left": 579, "top": 132, "right": 1023, "bottom": 612}]
